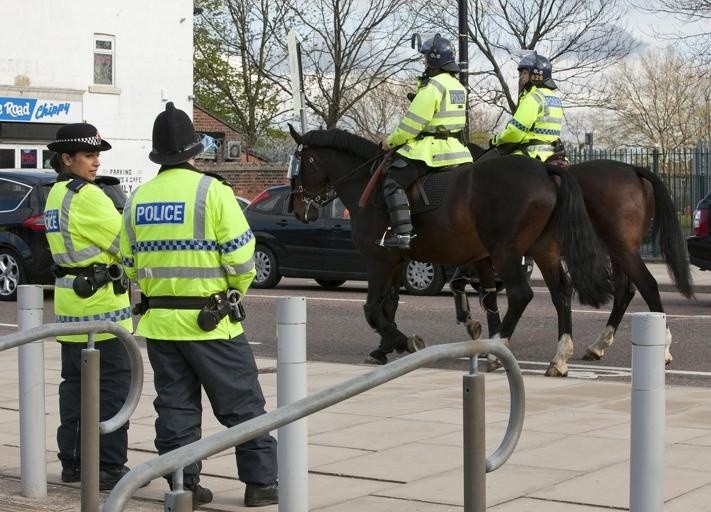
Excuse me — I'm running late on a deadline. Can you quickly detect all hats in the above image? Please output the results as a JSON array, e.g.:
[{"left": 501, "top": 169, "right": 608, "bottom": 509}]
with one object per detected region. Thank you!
[
  {"left": 148, "top": 102, "right": 203, "bottom": 165},
  {"left": 47, "top": 125, "right": 112, "bottom": 154}
]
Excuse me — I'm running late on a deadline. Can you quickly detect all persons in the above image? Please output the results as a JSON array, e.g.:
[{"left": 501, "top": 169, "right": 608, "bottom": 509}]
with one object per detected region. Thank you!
[
  {"left": 42, "top": 122, "right": 152, "bottom": 492},
  {"left": 118, "top": 100, "right": 280, "bottom": 509},
  {"left": 373, "top": 32, "right": 474, "bottom": 249},
  {"left": 487, "top": 49, "right": 568, "bottom": 165}
]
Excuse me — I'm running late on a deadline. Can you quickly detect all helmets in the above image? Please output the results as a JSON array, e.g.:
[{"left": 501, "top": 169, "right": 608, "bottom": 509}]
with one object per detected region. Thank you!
[
  {"left": 419, "top": 34, "right": 460, "bottom": 74},
  {"left": 517, "top": 51, "right": 558, "bottom": 90}
]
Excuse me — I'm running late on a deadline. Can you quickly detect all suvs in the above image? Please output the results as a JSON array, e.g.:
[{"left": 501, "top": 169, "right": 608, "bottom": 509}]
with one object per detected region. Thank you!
[{"left": 1, "top": 169, "right": 128, "bottom": 302}]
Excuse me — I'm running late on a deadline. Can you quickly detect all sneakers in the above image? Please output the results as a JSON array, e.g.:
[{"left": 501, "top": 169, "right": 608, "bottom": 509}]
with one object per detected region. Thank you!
[
  {"left": 94, "top": 466, "right": 151, "bottom": 491},
  {"left": 243, "top": 479, "right": 278, "bottom": 508},
  {"left": 169, "top": 481, "right": 213, "bottom": 511},
  {"left": 60, "top": 464, "right": 83, "bottom": 483}
]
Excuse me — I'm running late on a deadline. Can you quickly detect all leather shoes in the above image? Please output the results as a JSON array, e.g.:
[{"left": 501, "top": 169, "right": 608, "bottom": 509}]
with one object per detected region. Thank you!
[{"left": 374, "top": 234, "right": 412, "bottom": 249}]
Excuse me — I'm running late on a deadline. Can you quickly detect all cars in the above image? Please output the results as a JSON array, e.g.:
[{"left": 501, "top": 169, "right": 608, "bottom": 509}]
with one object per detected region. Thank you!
[
  {"left": 234, "top": 196, "right": 251, "bottom": 210},
  {"left": 686, "top": 191, "right": 711, "bottom": 271},
  {"left": 243, "top": 184, "right": 534, "bottom": 296}
]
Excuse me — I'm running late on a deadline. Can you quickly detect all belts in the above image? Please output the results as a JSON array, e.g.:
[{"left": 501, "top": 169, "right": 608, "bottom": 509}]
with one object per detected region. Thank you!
[
  {"left": 414, "top": 131, "right": 459, "bottom": 138},
  {"left": 520, "top": 138, "right": 547, "bottom": 148},
  {"left": 147, "top": 294, "right": 210, "bottom": 310},
  {"left": 52, "top": 262, "right": 96, "bottom": 278}
]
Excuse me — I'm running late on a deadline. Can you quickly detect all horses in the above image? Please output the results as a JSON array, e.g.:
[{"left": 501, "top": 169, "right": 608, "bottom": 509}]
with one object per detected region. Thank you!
[
  {"left": 407, "top": 92, "right": 693, "bottom": 365},
  {"left": 286, "top": 122, "right": 615, "bottom": 378}
]
